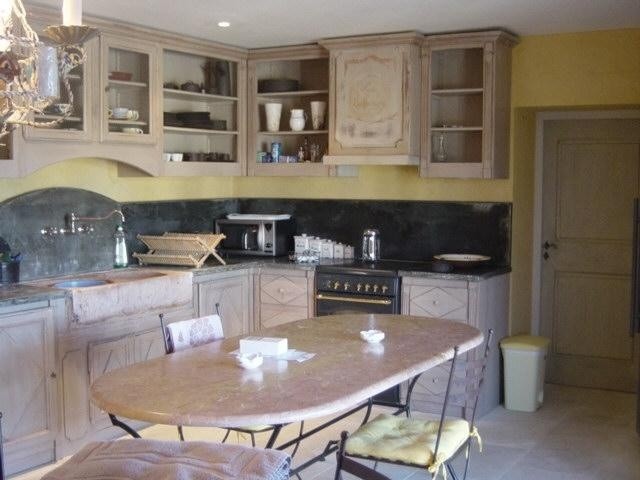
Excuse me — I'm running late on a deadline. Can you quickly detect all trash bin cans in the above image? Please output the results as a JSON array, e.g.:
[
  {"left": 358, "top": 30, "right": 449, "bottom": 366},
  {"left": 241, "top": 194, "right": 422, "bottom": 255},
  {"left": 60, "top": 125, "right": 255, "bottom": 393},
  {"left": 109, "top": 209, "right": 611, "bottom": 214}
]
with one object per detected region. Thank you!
[{"left": 499, "top": 334, "right": 551, "bottom": 413}]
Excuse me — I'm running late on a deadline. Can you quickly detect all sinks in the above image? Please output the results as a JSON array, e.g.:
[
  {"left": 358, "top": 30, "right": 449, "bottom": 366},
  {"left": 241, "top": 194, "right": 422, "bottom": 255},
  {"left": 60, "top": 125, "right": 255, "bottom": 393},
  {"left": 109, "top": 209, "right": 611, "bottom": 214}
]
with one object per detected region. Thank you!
[
  {"left": 98, "top": 266, "right": 193, "bottom": 316},
  {"left": 18, "top": 275, "right": 118, "bottom": 325}
]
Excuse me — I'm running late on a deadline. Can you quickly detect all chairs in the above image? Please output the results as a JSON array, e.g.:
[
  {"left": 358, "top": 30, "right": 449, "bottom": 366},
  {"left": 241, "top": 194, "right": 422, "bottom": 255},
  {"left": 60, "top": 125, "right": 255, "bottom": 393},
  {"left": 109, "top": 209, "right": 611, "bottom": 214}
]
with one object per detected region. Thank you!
[
  {"left": 158, "top": 302, "right": 294, "bottom": 449},
  {"left": 332, "top": 328, "right": 495, "bottom": 480}
]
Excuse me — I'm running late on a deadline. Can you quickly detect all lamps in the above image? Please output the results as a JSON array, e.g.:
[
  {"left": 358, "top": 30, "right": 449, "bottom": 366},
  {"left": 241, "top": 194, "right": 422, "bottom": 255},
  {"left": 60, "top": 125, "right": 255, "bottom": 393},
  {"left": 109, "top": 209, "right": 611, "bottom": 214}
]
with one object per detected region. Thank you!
[{"left": 0, "top": 0, "right": 100, "bottom": 142}]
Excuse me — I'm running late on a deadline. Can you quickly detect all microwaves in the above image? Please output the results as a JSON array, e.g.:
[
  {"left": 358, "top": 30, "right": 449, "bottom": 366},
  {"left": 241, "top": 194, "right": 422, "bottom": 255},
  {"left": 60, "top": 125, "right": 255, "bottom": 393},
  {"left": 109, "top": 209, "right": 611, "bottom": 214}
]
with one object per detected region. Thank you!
[{"left": 215, "top": 217, "right": 296, "bottom": 257}]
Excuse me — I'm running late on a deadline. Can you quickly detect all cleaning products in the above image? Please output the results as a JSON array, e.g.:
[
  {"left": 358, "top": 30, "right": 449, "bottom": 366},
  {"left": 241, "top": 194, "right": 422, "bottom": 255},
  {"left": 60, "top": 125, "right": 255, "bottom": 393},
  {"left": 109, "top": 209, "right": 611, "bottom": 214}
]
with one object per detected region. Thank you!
[{"left": 112, "top": 225, "right": 130, "bottom": 268}]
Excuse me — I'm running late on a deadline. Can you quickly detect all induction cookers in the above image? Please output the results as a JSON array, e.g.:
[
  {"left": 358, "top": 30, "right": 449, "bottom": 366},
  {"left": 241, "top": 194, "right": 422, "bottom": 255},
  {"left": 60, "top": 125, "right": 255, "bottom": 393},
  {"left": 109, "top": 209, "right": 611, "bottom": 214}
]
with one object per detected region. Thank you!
[{"left": 331, "top": 258, "right": 433, "bottom": 273}]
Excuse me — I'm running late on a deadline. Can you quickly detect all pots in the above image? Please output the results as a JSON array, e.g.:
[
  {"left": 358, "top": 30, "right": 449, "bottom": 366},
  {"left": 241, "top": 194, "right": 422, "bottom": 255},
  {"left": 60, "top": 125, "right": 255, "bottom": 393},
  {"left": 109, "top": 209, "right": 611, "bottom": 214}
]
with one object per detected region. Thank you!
[{"left": 113, "top": 108, "right": 128, "bottom": 118}]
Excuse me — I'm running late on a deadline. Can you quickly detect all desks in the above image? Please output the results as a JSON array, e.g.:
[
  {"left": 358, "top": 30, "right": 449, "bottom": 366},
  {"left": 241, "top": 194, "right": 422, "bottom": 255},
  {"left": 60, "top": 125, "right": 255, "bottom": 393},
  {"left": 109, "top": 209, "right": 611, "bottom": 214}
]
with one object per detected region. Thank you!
[{"left": 91, "top": 313, "right": 485, "bottom": 480}]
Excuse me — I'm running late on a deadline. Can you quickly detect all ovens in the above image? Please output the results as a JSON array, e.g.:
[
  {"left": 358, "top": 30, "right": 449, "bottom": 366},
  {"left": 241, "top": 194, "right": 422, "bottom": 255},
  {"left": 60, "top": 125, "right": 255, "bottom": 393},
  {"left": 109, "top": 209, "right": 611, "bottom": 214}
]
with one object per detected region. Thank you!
[{"left": 314, "top": 272, "right": 399, "bottom": 317}]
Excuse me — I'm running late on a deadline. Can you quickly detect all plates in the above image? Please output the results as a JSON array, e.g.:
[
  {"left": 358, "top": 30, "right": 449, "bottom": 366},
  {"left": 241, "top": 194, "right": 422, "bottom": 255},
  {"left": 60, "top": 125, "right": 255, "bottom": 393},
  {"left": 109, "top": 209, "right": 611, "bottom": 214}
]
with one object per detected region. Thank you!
[
  {"left": 183, "top": 151, "right": 231, "bottom": 162},
  {"left": 165, "top": 112, "right": 227, "bottom": 130},
  {"left": 257, "top": 78, "right": 300, "bottom": 93},
  {"left": 433, "top": 252, "right": 493, "bottom": 269}
]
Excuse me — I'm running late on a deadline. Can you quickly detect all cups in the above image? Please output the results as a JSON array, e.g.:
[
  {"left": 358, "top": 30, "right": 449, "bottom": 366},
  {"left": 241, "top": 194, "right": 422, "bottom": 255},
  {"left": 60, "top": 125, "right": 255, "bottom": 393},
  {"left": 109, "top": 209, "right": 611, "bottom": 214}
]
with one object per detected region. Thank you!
[
  {"left": 264, "top": 102, "right": 282, "bottom": 130},
  {"left": 310, "top": 101, "right": 327, "bottom": 130}
]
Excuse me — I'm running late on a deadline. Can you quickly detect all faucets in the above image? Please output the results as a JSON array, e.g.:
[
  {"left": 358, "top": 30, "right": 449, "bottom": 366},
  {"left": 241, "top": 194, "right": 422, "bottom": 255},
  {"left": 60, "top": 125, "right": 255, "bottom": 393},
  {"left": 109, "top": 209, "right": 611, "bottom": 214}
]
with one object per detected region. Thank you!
[{"left": 64, "top": 209, "right": 126, "bottom": 236}]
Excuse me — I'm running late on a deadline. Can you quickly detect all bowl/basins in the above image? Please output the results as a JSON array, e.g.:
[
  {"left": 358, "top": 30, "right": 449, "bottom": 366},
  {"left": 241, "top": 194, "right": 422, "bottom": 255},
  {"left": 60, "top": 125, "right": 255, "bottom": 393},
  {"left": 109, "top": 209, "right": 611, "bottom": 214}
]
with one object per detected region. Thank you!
[
  {"left": 112, "top": 72, "right": 133, "bottom": 80},
  {"left": 164, "top": 153, "right": 171, "bottom": 160},
  {"left": 54, "top": 103, "right": 74, "bottom": 117},
  {"left": 172, "top": 153, "right": 184, "bottom": 161}
]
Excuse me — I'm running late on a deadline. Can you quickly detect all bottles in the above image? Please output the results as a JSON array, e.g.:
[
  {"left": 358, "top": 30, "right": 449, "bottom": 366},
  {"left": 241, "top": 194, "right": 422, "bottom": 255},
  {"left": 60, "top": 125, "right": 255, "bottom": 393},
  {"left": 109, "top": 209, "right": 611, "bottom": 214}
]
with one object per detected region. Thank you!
[
  {"left": 296, "top": 137, "right": 317, "bottom": 162},
  {"left": 271, "top": 143, "right": 282, "bottom": 162},
  {"left": 293, "top": 233, "right": 354, "bottom": 260},
  {"left": 361, "top": 229, "right": 380, "bottom": 262},
  {"left": 434, "top": 135, "right": 448, "bottom": 162},
  {"left": 114, "top": 226, "right": 128, "bottom": 270}
]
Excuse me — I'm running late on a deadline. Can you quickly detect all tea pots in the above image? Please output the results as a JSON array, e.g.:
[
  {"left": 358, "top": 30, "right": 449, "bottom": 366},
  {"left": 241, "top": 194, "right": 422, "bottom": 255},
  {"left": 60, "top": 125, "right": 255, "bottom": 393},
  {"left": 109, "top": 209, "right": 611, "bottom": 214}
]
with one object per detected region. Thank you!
[
  {"left": 163, "top": 80, "right": 179, "bottom": 90},
  {"left": 289, "top": 109, "right": 308, "bottom": 130},
  {"left": 108, "top": 107, "right": 113, "bottom": 119},
  {"left": 127, "top": 110, "right": 139, "bottom": 120},
  {"left": 183, "top": 82, "right": 201, "bottom": 92},
  {"left": 121, "top": 127, "right": 143, "bottom": 134}
]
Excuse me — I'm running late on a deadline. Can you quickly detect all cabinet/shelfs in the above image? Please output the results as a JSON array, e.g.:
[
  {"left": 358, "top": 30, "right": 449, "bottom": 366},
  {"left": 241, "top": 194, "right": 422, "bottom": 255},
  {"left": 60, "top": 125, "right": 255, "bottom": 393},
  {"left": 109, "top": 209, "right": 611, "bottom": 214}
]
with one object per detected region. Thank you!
[
  {"left": 194, "top": 270, "right": 253, "bottom": 340},
  {"left": 399, "top": 283, "right": 477, "bottom": 410},
  {"left": 162, "top": 38, "right": 244, "bottom": 171},
  {"left": 250, "top": 50, "right": 332, "bottom": 175},
  {"left": 25, "top": 23, "right": 160, "bottom": 147},
  {"left": 56, "top": 324, "right": 199, "bottom": 447},
  {"left": 0, "top": 304, "right": 58, "bottom": 480},
  {"left": 254, "top": 265, "right": 312, "bottom": 331},
  {"left": 419, "top": 27, "right": 510, "bottom": 181}
]
